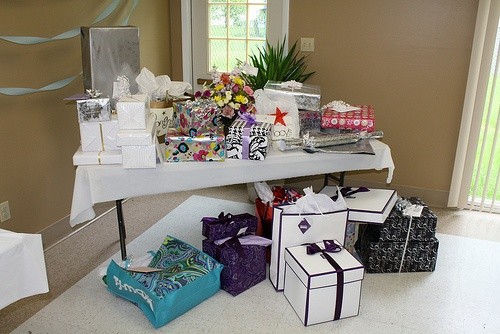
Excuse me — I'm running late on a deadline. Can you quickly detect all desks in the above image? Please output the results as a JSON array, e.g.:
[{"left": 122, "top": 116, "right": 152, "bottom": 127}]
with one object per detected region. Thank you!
[
  {"left": 69, "top": 138, "right": 396, "bottom": 261},
  {"left": 0, "top": 228, "right": 49, "bottom": 311}
]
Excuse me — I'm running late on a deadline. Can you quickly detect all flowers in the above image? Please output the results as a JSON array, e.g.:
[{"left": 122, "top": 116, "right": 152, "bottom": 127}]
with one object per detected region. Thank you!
[{"left": 194, "top": 69, "right": 257, "bottom": 119}]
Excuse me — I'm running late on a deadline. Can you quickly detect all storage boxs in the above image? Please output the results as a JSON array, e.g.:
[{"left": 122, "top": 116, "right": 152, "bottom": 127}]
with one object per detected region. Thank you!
[
  {"left": 165, "top": 124, "right": 226, "bottom": 162},
  {"left": 225, "top": 134, "right": 271, "bottom": 160},
  {"left": 201, "top": 213, "right": 259, "bottom": 241},
  {"left": 115, "top": 94, "right": 150, "bottom": 130},
  {"left": 76, "top": 96, "right": 112, "bottom": 124},
  {"left": 172, "top": 101, "right": 222, "bottom": 136},
  {"left": 353, "top": 223, "right": 439, "bottom": 275},
  {"left": 316, "top": 184, "right": 398, "bottom": 225},
  {"left": 116, "top": 114, "right": 157, "bottom": 147},
  {"left": 366, "top": 197, "right": 438, "bottom": 242},
  {"left": 282, "top": 239, "right": 365, "bottom": 327},
  {"left": 79, "top": 25, "right": 141, "bottom": 112},
  {"left": 148, "top": 106, "right": 175, "bottom": 138},
  {"left": 78, "top": 109, "right": 122, "bottom": 151},
  {"left": 121, "top": 134, "right": 158, "bottom": 170},
  {"left": 72, "top": 145, "right": 122, "bottom": 165},
  {"left": 320, "top": 104, "right": 376, "bottom": 132},
  {"left": 264, "top": 79, "right": 321, "bottom": 112},
  {"left": 254, "top": 114, "right": 301, "bottom": 141}
]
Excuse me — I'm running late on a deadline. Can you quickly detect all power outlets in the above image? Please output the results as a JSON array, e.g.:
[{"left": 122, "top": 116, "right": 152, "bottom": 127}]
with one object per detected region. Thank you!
[{"left": 0, "top": 201, "right": 12, "bottom": 223}]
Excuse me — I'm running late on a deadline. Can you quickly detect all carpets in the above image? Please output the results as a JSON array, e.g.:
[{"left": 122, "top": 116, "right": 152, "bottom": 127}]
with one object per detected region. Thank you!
[{"left": 5, "top": 194, "right": 500, "bottom": 334}]
